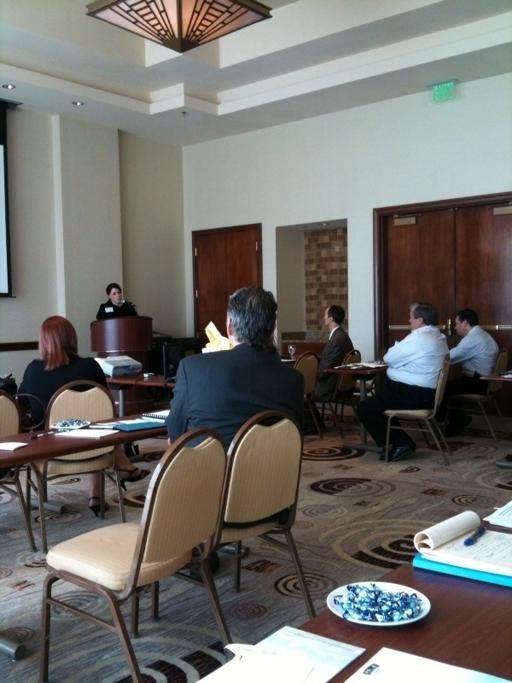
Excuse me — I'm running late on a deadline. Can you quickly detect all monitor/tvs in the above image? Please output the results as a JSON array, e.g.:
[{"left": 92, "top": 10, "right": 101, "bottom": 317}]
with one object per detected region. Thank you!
[
  {"left": 334, "top": 362, "right": 388, "bottom": 370},
  {"left": 413, "top": 511, "right": 511, "bottom": 590},
  {"left": 55, "top": 410, "right": 171, "bottom": 439}
]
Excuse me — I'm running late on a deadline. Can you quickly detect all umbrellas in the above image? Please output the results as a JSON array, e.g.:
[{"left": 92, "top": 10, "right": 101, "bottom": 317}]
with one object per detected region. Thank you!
[{"left": 378, "top": 439, "right": 415, "bottom": 461}]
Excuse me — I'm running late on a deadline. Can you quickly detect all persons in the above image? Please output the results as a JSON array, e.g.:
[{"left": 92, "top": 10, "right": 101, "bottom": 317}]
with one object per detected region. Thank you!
[
  {"left": 94, "top": 283, "right": 140, "bottom": 320},
  {"left": 305, "top": 305, "right": 355, "bottom": 435},
  {"left": 17, "top": 315, "right": 152, "bottom": 517},
  {"left": 353, "top": 301, "right": 451, "bottom": 462},
  {"left": 448, "top": 309, "right": 499, "bottom": 438},
  {"left": 166, "top": 286, "right": 306, "bottom": 583}
]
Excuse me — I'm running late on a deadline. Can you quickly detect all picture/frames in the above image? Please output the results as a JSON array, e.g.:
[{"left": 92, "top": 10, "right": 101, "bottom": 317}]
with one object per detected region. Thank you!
[
  {"left": 323, "top": 361, "right": 388, "bottom": 453},
  {"left": 90, "top": 317, "right": 155, "bottom": 365},
  {"left": 480, "top": 372, "right": 512, "bottom": 468},
  {"left": 106, "top": 362, "right": 321, "bottom": 416},
  {"left": 0, "top": 411, "right": 178, "bottom": 660},
  {"left": 195, "top": 496, "right": 512, "bottom": 683}
]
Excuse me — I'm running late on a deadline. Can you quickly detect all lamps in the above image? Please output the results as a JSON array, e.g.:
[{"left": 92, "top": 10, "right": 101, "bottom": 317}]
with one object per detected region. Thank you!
[
  {"left": 39, "top": 426, "right": 232, "bottom": 682},
  {"left": 292, "top": 352, "right": 323, "bottom": 440},
  {"left": 383, "top": 353, "right": 448, "bottom": 466},
  {"left": 27, "top": 378, "right": 126, "bottom": 554},
  {"left": 150, "top": 409, "right": 316, "bottom": 662},
  {"left": 313, "top": 350, "right": 363, "bottom": 439},
  {"left": 443, "top": 351, "right": 508, "bottom": 441},
  {"left": 1, "top": 392, "right": 36, "bottom": 553}
]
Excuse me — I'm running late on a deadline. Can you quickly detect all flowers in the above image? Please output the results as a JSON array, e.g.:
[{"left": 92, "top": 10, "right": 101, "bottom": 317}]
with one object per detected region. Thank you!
[{"left": 121, "top": 299, "right": 134, "bottom": 312}]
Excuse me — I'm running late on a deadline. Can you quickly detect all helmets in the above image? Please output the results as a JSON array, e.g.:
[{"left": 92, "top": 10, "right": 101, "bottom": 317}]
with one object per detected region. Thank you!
[
  {"left": 325, "top": 579, "right": 433, "bottom": 628},
  {"left": 50, "top": 419, "right": 92, "bottom": 430}
]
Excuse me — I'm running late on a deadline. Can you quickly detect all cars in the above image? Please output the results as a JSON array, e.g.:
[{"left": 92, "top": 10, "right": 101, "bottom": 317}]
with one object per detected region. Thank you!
[{"left": 287, "top": 344, "right": 295, "bottom": 359}]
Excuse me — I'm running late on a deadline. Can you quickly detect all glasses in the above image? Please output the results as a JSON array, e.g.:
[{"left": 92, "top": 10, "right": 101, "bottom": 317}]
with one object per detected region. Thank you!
[{"left": 84, "top": 0, "right": 275, "bottom": 54}]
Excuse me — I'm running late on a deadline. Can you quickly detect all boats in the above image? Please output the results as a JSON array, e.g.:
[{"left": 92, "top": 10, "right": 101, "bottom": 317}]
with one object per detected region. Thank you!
[{"left": 163, "top": 337, "right": 206, "bottom": 383}]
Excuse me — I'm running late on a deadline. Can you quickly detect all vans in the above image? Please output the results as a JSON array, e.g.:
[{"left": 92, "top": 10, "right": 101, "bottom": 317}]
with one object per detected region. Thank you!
[
  {"left": 88, "top": 496, "right": 111, "bottom": 518},
  {"left": 119, "top": 467, "right": 152, "bottom": 492}
]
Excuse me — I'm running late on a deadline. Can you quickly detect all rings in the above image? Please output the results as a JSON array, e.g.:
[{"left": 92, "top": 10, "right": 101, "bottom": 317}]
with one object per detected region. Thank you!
[
  {"left": 32, "top": 430, "right": 57, "bottom": 441},
  {"left": 465, "top": 524, "right": 486, "bottom": 545}
]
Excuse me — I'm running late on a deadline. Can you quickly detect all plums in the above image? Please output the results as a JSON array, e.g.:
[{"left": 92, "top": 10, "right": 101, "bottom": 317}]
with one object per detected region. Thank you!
[{"left": 93, "top": 356, "right": 143, "bottom": 377}]
